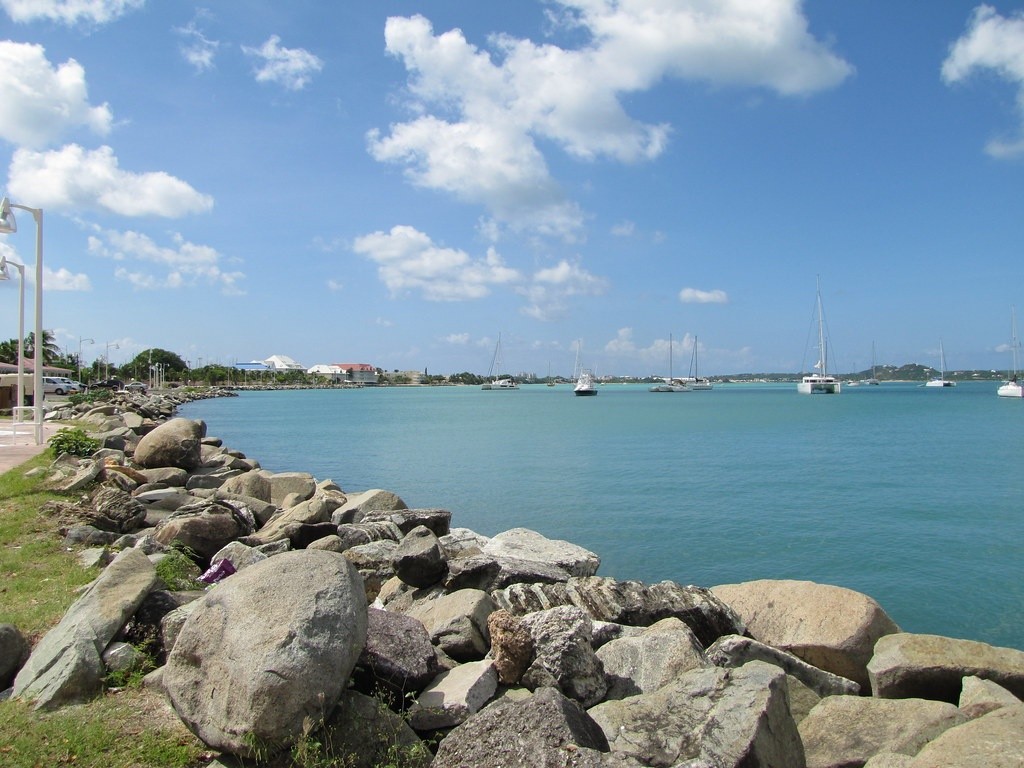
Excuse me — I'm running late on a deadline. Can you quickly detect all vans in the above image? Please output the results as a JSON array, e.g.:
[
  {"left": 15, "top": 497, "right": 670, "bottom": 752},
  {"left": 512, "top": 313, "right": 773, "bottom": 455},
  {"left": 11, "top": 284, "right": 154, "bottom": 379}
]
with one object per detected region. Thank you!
[
  {"left": 42, "top": 377, "right": 71, "bottom": 394},
  {"left": 0, "top": 373, "right": 46, "bottom": 406}
]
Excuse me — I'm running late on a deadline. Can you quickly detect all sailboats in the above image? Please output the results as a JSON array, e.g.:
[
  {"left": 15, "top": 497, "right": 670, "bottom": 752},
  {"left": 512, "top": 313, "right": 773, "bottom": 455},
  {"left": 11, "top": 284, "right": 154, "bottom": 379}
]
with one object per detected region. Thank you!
[
  {"left": 546, "top": 361, "right": 555, "bottom": 387},
  {"left": 648, "top": 331, "right": 694, "bottom": 392},
  {"left": 797, "top": 273, "right": 841, "bottom": 394},
  {"left": 480, "top": 332, "right": 520, "bottom": 391},
  {"left": 997, "top": 304, "right": 1024, "bottom": 398},
  {"left": 684, "top": 335, "right": 713, "bottom": 390},
  {"left": 925, "top": 338, "right": 956, "bottom": 388},
  {"left": 845, "top": 362, "right": 860, "bottom": 387},
  {"left": 864, "top": 340, "right": 880, "bottom": 386}
]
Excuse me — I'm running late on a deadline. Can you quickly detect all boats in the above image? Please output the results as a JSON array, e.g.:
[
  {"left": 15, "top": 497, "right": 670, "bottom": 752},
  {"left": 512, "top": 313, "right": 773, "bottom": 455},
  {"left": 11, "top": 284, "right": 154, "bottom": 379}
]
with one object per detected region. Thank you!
[{"left": 575, "top": 368, "right": 599, "bottom": 396}]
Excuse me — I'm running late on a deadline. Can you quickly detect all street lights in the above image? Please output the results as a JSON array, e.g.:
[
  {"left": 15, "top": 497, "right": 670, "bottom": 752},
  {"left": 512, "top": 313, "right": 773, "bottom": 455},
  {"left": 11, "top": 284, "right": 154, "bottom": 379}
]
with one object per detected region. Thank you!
[
  {"left": 79, "top": 336, "right": 95, "bottom": 385},
  {"left": 0, "top": 195, "right": 45, "bottom": 448},
  {"left": 106, "top": 341, "right": 119, "bottom": 384},
  {"left": 147, "top": 350, "right": 170, "bottom": 388}
]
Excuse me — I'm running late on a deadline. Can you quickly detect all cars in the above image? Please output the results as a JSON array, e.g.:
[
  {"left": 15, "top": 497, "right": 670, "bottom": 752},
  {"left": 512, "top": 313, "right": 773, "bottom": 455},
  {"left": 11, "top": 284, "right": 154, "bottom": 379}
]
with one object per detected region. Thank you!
[{"left": 60, "top": 376, "right": 148, "bottom": 392}]
